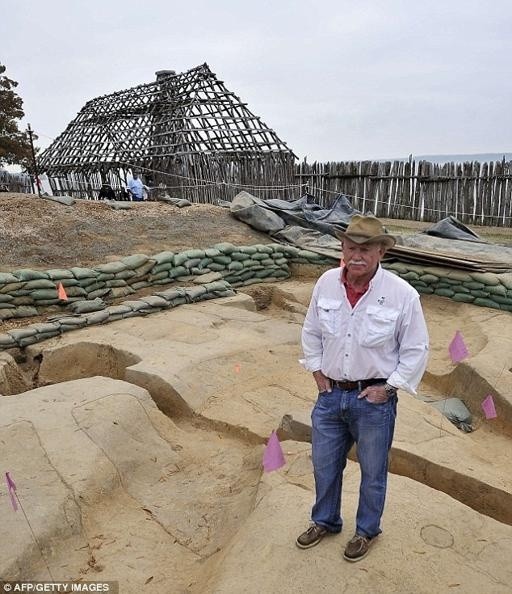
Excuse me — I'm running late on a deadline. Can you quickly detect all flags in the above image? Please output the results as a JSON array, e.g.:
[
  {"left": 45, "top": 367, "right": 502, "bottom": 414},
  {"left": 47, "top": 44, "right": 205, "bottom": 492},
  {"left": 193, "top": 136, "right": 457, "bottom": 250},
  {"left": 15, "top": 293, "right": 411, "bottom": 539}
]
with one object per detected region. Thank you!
[
  {"left": 263, "top": 431, "right": 285, "bottom": 473},
  {"left": 57, "top": 282, "right": 67, "bottom": 302},
  {"left": 481, "top": 394, "right": 497, "bottom": 420},
  {"left": 6, "top": 472, "right": 17, "bottom": 512},
  {"left": 448, "top": 330, "right": 470, "bottom": 362}
]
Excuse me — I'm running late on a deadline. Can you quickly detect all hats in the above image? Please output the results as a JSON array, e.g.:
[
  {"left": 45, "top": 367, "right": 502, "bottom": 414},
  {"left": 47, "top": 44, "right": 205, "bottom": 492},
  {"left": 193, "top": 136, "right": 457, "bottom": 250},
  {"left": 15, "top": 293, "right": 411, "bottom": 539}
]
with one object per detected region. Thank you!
[{"left": 332, "top": 214, "right": 396, "bottom": 250}]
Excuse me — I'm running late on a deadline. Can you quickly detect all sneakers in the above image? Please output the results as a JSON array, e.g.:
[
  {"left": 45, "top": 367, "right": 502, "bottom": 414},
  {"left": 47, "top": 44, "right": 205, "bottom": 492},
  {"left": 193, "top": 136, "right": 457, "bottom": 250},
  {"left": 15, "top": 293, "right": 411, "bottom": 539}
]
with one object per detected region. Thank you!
[
  {"left": 343, "top": 534, "right": 373, "bottom": 562},
  {"left": 296, "top": 524, "right": 327, "bottom": 549}
]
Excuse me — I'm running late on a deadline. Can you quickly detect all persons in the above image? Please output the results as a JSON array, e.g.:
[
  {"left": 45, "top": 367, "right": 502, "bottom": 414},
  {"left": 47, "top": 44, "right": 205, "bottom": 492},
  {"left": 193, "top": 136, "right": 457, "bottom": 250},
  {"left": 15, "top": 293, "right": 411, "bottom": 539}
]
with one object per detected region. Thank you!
[
  {"left": 296, "top": 214, "right": 429, "bottom": 562},
  {"left": 98, "top": 181, "right": 117, "bottom": 201},
  {"left": 128, "top": 173, "right": 149, "bottom": 200}
]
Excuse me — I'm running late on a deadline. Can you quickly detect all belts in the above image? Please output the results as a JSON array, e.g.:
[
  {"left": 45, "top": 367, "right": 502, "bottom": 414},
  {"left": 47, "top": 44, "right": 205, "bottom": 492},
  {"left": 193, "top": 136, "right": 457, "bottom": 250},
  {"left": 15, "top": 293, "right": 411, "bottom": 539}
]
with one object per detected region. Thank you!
[{"left": 329, "top": 378, "right": 387, "bottom": 390}]
